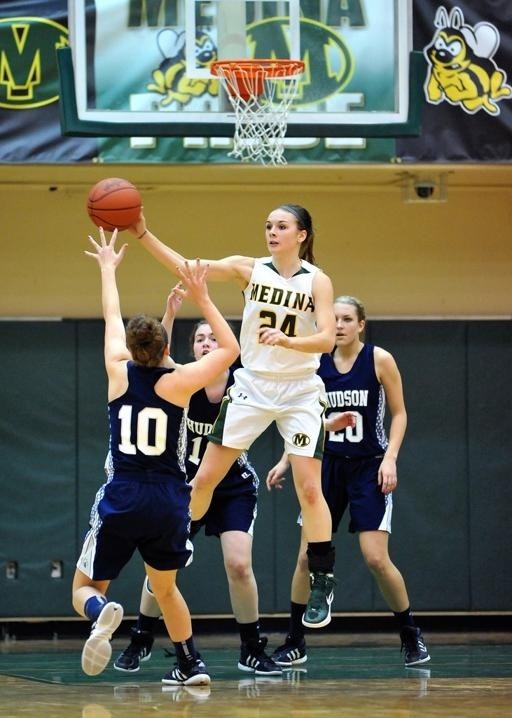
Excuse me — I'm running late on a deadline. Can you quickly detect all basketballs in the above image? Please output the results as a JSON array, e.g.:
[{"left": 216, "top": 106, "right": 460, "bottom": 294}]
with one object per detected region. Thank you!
[{"left": 87, "top": 177, "right": 141, "bottom": 230}]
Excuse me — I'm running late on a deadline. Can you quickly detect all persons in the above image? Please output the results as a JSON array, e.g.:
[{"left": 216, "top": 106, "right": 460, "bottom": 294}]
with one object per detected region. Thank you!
[
  {"left": 120, "top": 203, "right": 336, "bottom": 630},
  {"left": 70, "top": 224, "right": 240, "bottom": 690},
  {"left": 265, "top": 294, "right": 431, "bottom": 669},
  {"left": 112, "top": 279, "right": 284, "bottom": 677}
]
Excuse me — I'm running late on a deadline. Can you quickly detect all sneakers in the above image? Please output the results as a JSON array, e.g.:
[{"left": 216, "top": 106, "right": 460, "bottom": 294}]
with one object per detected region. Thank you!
[
  {"left": 399, "top": 625, "right": 431, "bottom": 666},
  {"left": 268, "top": 632, "right": 308, "bottom": 667},
  {"left": 160, "top": 656, "right": 210, "bottom": 684},
  {"left": 237, "top": 636, "right": 280, "bottom": 675},
  {"left": 112, "top": 626, "right": 155, "bottom": 671},
  {"left": 81, "top": 602, "right": 124, "bottom": 676},
  {"left": 301, "top": 572, "right": 336, "bottom": 630}
]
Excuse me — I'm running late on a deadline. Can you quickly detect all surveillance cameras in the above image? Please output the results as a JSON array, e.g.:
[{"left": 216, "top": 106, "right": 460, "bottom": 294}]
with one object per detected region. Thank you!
[{"left": 413, "top": 177, "right": 437, "bottom": 200}]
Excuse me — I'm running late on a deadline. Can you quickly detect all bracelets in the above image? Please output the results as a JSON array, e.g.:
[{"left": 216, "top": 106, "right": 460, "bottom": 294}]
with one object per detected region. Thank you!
[{"left": 137, "top": 231, "right": 147, "bottom": 239}]
